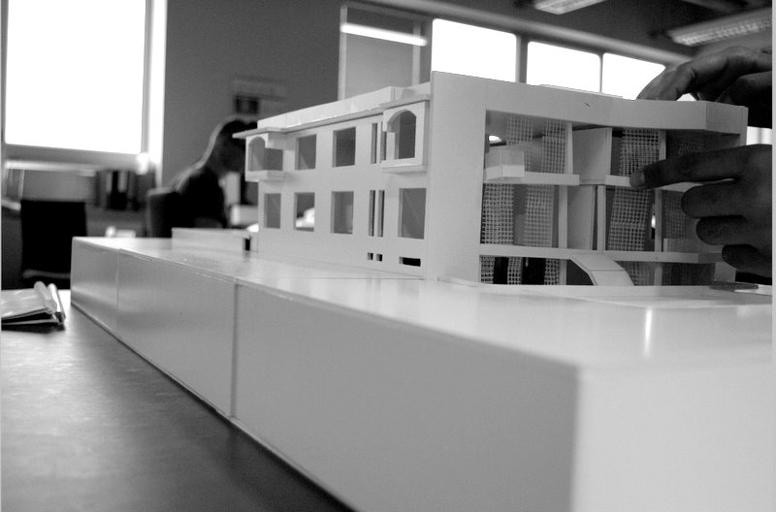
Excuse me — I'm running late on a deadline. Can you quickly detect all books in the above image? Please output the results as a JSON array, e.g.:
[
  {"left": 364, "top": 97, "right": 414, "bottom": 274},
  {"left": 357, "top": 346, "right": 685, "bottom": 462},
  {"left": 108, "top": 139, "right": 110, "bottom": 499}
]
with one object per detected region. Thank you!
[{"left": 1, "top": 279, "right": 67, "bottom": 329}]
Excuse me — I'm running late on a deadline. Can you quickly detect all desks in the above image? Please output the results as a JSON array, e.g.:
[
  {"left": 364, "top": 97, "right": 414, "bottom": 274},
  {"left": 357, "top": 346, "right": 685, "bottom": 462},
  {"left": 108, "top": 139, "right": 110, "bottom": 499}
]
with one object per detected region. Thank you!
[
  {"left": 0, "top": 196, "right": 144, "bottom": 225},
  {"left": 1, "top": 280, "right": 351, "bottom": 510}
]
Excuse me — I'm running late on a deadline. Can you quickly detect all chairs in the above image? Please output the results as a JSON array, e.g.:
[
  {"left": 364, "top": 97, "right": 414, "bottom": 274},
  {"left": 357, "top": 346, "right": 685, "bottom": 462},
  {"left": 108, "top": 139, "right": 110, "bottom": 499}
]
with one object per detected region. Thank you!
[
  {"left": 143, "top": 186, "right": 173, "bottom": 238},
  {"left": 19, "top": 196, "right": 90, "bottom": 289}
]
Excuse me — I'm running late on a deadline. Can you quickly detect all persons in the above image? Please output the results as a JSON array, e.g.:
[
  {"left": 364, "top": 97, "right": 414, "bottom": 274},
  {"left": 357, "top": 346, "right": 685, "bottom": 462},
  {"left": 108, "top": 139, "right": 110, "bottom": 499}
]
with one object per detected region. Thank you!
[
  {"left": 628, "top": 41, "right": 771, "bottom": 282},
  {"left": 168, "top": 115, "right": 262, "bottom": 243}
]
